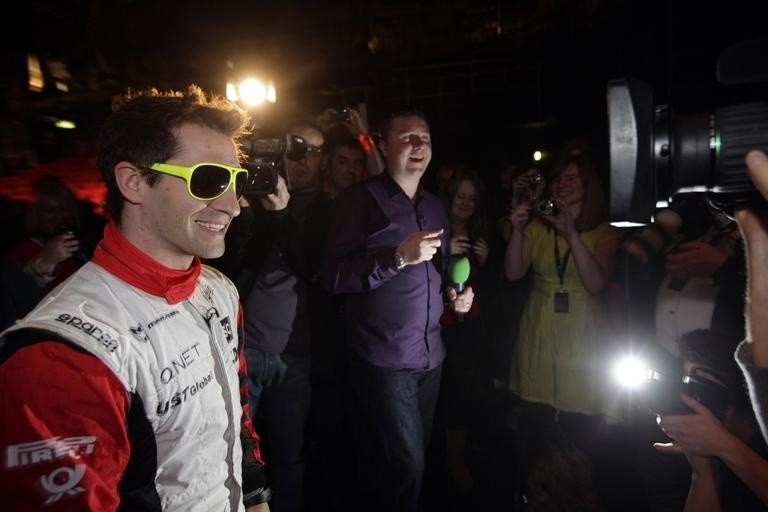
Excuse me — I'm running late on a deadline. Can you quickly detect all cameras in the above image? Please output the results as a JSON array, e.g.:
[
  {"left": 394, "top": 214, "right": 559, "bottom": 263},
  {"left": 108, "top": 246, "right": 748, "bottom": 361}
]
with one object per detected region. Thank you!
[
  {"left": 528, "top": 174, "right": 540, "bottom": 183},
  {"left": 533, "top": 200, "right": 558, "bottom": 216},
  {"left": 330, "top": 109, "right": 350, "bottom": 123},
  {"left": 602, "top": 77, "right": 768, "bottom": 228},
  {"left": 631, "top": 354, "right": 729, "bottom": 431}
]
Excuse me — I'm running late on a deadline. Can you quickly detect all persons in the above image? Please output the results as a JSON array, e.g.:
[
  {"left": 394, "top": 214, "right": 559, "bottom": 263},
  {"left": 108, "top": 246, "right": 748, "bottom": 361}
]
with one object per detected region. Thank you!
[
  {"left": 207, "top": 104, "right": 766, "bottom": 511},
  {"left": 0, "top": 83, "right": 270, "bottom": 512},
  {"left": 3, "top": 172, "right": 106, "bottom": 320}
]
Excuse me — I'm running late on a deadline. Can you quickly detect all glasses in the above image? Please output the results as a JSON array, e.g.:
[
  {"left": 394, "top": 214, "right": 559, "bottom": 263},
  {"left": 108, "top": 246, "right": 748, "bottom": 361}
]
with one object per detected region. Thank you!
[{"left": 136, "top": 161, "right": 249, "bottom": 201}]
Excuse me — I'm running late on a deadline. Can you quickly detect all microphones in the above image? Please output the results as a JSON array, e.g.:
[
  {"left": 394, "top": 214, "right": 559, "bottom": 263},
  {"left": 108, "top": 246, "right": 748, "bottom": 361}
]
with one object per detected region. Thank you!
[{"left": 447, "top": 255, "right": 472, "bottom": 322}]
